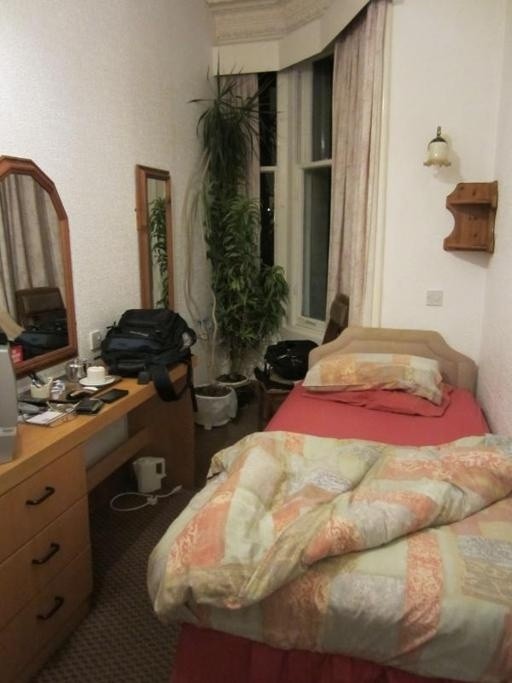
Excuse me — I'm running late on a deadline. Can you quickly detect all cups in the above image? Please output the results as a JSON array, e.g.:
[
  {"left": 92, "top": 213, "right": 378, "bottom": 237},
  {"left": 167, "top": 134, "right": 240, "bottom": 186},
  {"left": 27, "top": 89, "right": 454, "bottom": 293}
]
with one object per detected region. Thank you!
[
  {"left": 85, "top": 365, "right": 105, "bottom": 383},
  {"left": 64, "top": 358, "right": 85, "bottom": 382},
  {"left": 30, "top": 382, "right": 50, "bottom": 398}
]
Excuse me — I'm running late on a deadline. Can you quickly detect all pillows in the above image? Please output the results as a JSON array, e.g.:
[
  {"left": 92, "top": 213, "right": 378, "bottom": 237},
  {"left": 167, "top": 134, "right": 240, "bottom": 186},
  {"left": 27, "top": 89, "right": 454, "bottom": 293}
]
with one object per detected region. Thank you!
[
  {"left": 301, "top": 352, "right": 444, "bottom": 406},
  {"left": 300, "top": 383, "right": 459, "bottom": 417}
]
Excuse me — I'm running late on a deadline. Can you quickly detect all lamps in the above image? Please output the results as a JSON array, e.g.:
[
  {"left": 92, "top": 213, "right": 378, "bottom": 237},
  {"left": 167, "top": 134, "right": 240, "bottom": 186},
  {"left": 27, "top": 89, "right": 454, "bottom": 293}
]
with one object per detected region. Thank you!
[{"left": 423, "top": 125, "right": 452, "bottom": 168}]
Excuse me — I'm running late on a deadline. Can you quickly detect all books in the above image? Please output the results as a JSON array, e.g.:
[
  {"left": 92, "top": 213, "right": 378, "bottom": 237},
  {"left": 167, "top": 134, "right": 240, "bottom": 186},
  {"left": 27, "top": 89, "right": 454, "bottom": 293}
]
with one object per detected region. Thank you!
[{"left": 17, "top": 401, "right": 76, "bottom": 427}]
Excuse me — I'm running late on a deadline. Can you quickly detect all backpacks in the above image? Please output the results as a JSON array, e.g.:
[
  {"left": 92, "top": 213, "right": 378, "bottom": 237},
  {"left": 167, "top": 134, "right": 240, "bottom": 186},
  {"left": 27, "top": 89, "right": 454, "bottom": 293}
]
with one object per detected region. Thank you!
[{"left": 101, "top": 307, "right": 200, "bottom": 413}]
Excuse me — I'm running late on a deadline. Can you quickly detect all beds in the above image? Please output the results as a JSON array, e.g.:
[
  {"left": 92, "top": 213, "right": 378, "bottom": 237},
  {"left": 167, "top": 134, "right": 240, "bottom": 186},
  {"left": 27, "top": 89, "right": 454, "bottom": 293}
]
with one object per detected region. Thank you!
[{"left": 146, "top": 326, "right": 512, "bottom": 683}]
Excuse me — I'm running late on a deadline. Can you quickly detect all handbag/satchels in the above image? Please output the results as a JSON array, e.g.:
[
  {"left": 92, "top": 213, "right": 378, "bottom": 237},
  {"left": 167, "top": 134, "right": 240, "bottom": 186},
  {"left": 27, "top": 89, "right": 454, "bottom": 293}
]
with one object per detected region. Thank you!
[{"left": 264, "top": 340, "right": 318, "bottom": 382}]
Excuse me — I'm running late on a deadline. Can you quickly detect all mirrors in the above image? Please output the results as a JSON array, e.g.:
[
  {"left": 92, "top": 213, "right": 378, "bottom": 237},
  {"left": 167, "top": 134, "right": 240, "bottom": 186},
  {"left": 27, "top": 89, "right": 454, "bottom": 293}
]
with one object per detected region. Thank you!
[
  {"left": 135, "top": 164, "right": 174, "bottom": 312},
  {"left": 0, "top": 155, "right": 79, "bottom": 380}
]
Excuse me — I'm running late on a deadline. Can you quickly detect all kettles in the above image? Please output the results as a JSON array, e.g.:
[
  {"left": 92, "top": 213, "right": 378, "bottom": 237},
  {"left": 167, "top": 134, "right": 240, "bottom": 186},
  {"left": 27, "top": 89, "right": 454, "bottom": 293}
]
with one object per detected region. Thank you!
[{"left": 131, "top": 455, "right": 167, "bottom": 493}]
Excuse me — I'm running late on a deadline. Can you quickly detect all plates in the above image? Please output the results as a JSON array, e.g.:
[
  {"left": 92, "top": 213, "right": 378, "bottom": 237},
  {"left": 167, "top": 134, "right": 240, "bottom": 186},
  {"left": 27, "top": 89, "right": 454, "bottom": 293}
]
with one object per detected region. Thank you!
[{"left": 78, "top": 374, "right": 116, "bottom": 386}]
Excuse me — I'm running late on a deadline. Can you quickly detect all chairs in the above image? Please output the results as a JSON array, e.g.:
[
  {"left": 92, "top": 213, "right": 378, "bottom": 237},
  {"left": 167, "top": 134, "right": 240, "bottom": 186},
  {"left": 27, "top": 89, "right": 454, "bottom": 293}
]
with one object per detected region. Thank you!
[{"left": 253, "top": 293, "right": 350, "bottom": 429}]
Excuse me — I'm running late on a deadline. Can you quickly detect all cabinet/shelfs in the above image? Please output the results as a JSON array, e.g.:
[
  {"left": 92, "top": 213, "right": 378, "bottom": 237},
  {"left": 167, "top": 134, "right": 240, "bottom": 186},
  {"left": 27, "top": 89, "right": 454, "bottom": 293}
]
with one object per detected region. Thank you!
[
  {"left": 442, "top": 180, "right": 499, "bottom": 254},
  {"left": 0, "top": 353, "right": 198, "bottom": 683}
]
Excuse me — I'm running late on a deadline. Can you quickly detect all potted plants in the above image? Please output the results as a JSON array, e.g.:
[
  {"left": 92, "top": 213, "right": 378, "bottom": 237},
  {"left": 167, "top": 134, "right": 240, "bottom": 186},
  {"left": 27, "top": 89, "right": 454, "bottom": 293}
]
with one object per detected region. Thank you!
[
  {"left": 185, "top": 279, "right": 239, "bottom": 431},
  {"left": 186, "top": 52, "right": 288, "bottom": 406}
]
